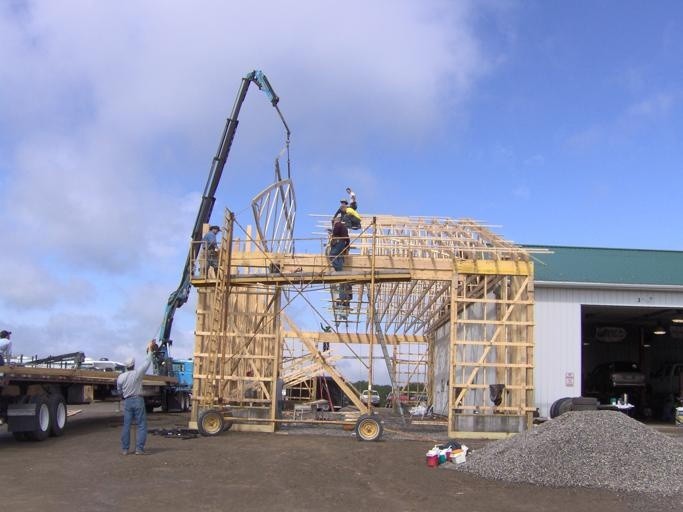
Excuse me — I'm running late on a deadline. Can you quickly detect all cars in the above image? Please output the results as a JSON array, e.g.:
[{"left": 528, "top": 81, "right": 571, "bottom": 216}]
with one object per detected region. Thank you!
[{"left": 584, "top": 360, "right": 650, "bottom": 394}]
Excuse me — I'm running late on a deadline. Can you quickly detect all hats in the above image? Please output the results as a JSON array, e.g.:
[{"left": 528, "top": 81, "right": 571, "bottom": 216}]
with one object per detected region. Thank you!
[
  {"left": 0, "top": 331, "right": 11, "bottom": 338},
  {"left": 125, "top": 358, "right": 135, "bottom": 368},
  {"left": 340, "top": 199, "right": 348, "bottom": 204},
  {"left": 210, "top": 226, "right": 221, "bottom": 231}
]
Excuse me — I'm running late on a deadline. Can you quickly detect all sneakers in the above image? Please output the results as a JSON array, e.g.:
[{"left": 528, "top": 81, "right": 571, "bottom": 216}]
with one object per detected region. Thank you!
[{"left": 120, "top": 448, "right": 145, "bottom": 455}]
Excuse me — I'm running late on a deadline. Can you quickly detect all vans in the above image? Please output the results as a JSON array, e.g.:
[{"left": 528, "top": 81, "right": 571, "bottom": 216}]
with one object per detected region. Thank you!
[
  {"left": 359, "top": 390, "right": 381, "bottom": 407},
  {"left": 386, "top": 391, "right": 409, "bottom": 407}
]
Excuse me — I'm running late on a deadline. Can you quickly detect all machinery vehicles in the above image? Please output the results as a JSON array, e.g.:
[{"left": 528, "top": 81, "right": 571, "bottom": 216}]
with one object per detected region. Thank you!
[{"left": 0, "top": 68, "right": 294, "bottom": 441}]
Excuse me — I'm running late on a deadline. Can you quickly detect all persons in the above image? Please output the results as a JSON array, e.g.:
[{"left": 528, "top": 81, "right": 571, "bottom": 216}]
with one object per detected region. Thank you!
[
  {"left": 319, "top": 322, "right": 332, "bottom": 352},
  {"left": 336, "top": 284, "right": 353, "bottom": 301},
  {"left": 199, "top": 225, "right": 221, "bottom": 279},
  {"left": 116, "top": 340, "right": 154, "bottom": 456},
  {"left": 332, "top": 199, "right": 349, "bottom": 221},
  {"left": 0, "top": 330, "right": 13, "bottom": 366},
  {"left": 339, "top": 205, "right": 361, "bottom": 231},
  {"left": 332, "top": 298, "right": 351, "bottom": 327},
  {"left": 329, "top": 215, "right": 351, "bottom": 269},
  {"left": 345, "top": 187, "right": 357, "bottom": 211},
  {"left": 146, "top": 339, "right": 158, "bottom": 355}
]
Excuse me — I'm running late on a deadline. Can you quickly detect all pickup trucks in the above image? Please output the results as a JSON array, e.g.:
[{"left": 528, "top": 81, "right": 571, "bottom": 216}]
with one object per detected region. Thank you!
[{"left": 647, "top": 362, "right": 682, "bottom": 396}]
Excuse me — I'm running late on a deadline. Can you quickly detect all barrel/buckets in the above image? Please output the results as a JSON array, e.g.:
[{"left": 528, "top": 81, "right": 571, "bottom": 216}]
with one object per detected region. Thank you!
[{"left": 675, "top": 407, "right": 683, "bottom": 425}]
